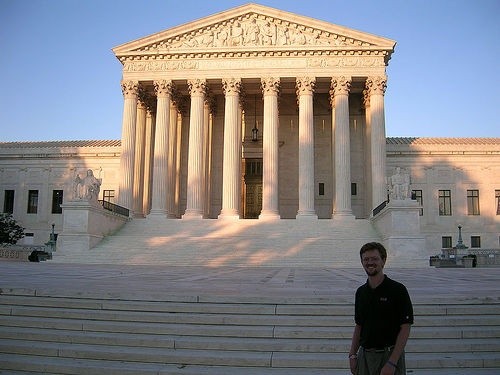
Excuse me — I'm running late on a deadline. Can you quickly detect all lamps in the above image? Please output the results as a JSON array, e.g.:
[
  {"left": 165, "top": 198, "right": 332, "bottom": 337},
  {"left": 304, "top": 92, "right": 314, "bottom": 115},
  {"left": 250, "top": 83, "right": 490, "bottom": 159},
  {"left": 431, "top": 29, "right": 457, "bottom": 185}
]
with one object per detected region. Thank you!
[{"left": 251, "top": 95, "right": 259, "bottom": 141}]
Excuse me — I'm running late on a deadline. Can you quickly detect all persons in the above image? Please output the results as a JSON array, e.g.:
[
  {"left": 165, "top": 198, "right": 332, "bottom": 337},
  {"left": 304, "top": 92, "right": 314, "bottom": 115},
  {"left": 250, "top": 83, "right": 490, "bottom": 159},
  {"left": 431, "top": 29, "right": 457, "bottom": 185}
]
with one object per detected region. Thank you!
[
  {"left": 391, "top": 165, "right": 412, "bottom": 199},
  {"left": 182, "top": 16, "right": 336, "bottom": 46},
  {"left": 75, "top": 169, "right": 100, "bottom": 199},
  {"left": 348, "top": 242, "right": 414, "bottom": 375}
]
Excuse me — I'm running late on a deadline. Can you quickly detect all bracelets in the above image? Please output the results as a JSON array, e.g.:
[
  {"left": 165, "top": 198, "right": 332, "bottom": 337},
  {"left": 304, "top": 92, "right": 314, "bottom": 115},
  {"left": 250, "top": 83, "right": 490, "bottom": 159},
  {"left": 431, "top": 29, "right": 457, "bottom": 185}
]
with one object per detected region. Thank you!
[
  {"left": 387, "top": 360, "right": 398, "bottom": 368},
  {"left": 349, "top": 354, "right": 358, "bottom": 359}
]
